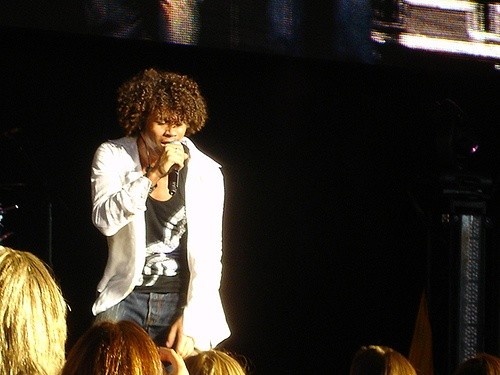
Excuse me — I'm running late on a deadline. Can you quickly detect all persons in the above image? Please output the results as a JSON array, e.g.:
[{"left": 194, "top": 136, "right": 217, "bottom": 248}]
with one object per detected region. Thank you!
[
  {"left": 90, "top": 68, "right": 232, "bottom": 372},
  {"left": 350, "top": 344, "right": 418, "bottom": 375},
  {"left": 0, "top": 244, "right": 69, "bottom": 375},
  {"left": 184, "top": 348, "right": 249, "bottom": 375},
  {"left": 455, "top": 352, "right": 500, "bottom": 375},
  {"left": 58, "top": 320, "right": 189, "bottom": 375}
]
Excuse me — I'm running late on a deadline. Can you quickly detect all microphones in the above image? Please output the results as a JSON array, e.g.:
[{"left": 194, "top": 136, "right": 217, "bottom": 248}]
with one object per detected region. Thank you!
[{"left": 168, "top": 140, "right": 181, "bottom": 194}]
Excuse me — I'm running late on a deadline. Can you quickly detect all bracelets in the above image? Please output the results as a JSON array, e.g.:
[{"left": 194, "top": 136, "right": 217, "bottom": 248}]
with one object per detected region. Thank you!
[{"left": 143, "top": 173, "right": 158, "bottom": 194}]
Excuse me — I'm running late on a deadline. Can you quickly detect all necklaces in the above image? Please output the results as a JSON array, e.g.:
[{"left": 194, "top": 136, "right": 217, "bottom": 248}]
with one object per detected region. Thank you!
[{"left": 145, "top": 163, "right": 153, "bottom": 173}]
